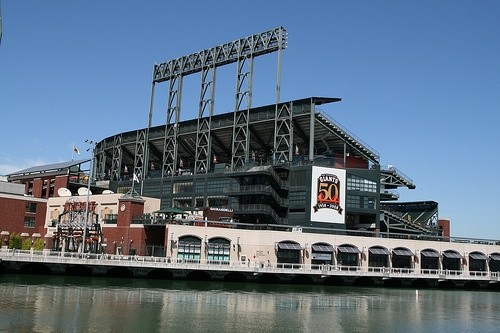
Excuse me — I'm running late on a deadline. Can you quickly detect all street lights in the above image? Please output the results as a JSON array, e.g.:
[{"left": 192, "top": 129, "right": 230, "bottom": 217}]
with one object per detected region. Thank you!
[{"left": 82, "top": 137, "right": 99, "bottom": 258}]
